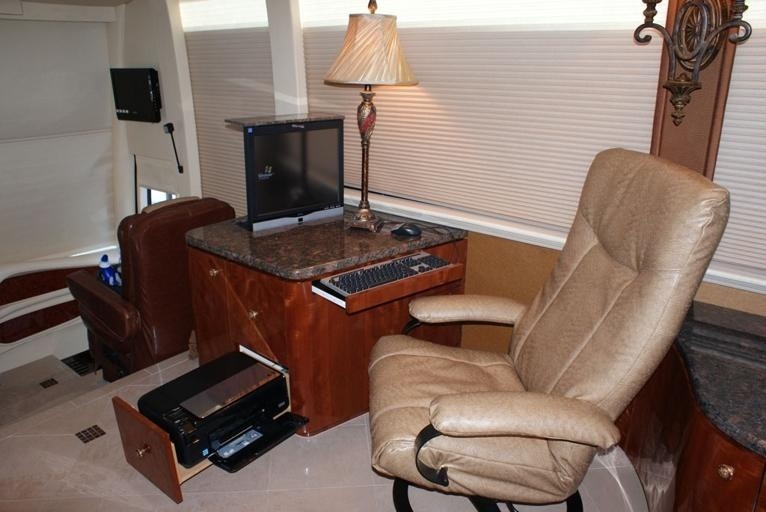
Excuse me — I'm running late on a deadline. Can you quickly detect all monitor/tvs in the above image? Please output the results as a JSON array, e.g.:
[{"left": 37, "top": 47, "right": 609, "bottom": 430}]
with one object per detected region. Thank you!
[
  {"left": 110, "top": 68, "right": 161, "bottom": 123},
  {"left": 235, "top": 120, "right": 344, "bottom": 232}
]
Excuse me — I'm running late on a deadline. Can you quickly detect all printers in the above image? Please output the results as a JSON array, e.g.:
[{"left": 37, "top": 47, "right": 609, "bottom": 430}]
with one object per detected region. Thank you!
[{"left": 137, "top": 350, "right": 310, "bottom": 474}]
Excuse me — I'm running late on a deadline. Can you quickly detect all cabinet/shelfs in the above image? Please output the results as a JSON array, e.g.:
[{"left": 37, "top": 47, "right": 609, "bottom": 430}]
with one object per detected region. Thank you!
[
  {"left": 665, "top": 289, "right": 766, "bottom": 507},
  {"left": 115, "top": 145, "right": 468, "bottom": 504}
]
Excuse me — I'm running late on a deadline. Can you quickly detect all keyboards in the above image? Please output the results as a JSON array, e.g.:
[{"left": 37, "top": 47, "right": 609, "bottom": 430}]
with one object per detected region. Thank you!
[{"left": 320, "top": 250, "right": 453, "bottom": 297}]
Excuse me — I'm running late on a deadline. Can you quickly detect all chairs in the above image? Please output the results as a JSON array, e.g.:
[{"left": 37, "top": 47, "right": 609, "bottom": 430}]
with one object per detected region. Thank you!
[
  {"left": 61, "top": 191, "right": 238, "bottom": 382},
  {"left": 364, "top": 148, "right": 733, "bottom": 512}
]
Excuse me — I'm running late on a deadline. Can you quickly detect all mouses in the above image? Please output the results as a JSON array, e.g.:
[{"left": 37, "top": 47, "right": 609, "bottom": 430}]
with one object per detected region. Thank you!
[{"left": 390, "top": 222, "right": 422, "bottom": 236}]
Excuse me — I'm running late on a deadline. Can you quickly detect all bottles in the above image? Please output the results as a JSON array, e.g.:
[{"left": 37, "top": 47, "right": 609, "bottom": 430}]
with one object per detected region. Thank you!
[{"left": 98, "top": 255, "right": 114, "bottom": 287}]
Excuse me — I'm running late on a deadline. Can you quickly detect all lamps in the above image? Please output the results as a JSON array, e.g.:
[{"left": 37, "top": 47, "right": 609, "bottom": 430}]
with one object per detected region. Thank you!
[{"left": 323, "top": 0, "right": 419, "bottom": 237}]
